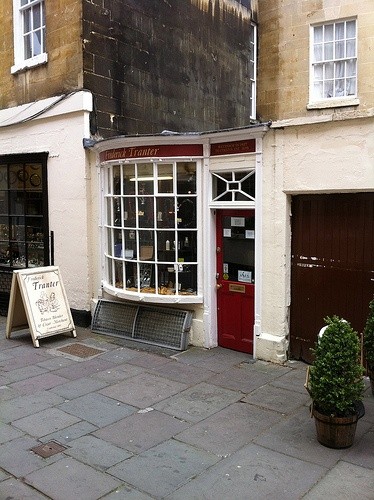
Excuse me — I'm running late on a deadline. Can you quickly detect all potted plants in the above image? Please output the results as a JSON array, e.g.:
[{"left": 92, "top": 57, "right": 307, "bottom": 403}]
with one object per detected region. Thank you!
[
  {"left": 365, "top": 297, "right": 374, "bottom": 395},
  {"left": 310, "top": 316, "right": 367, "bottom": 449}
]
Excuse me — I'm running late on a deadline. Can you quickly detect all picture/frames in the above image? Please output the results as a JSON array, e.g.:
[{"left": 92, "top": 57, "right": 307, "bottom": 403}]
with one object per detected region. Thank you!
[{"left": 133, "top": 258, "right": 155, "bottom": 288}]
